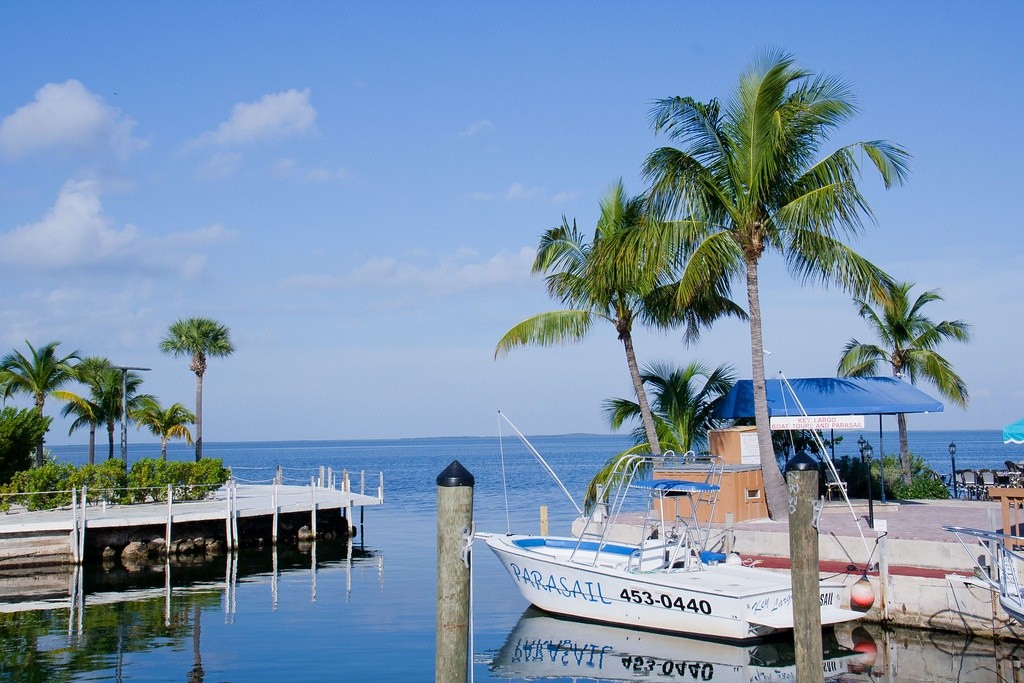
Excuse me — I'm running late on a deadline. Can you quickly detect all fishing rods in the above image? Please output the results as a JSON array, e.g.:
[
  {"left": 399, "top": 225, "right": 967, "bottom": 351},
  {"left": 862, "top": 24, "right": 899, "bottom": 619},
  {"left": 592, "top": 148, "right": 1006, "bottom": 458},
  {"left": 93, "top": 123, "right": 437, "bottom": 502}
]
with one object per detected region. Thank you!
[
  {"left": 498, "top": 410, "right": 598, "bottom": 541},
  {"left": 778, "top": 371, "right": 878, "bottom": 573}
]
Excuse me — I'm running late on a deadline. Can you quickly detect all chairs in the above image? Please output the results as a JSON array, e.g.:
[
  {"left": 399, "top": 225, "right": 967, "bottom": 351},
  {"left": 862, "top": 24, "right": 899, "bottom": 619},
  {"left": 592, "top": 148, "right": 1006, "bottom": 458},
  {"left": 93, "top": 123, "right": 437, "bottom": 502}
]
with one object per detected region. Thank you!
[
  {"left": 933, "top": 460, "right": 1024, "bottom": 501},
  {"left": 824, "top": 466, "right": 848, "bottom": 502}
]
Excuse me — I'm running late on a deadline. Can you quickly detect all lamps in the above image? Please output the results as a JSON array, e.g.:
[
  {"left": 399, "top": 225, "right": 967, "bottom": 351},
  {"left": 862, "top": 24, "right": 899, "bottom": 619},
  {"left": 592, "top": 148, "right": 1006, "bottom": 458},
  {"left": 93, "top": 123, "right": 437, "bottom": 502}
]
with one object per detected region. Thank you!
[
  {"left": 862, "top": 440, "right": 874, "bottom": 460},
  {"left": 948, "top": 440, "right": 957, "bottom": 455},
  {"left": 857, "top": 435, "right": 866, "bottom": 452}
]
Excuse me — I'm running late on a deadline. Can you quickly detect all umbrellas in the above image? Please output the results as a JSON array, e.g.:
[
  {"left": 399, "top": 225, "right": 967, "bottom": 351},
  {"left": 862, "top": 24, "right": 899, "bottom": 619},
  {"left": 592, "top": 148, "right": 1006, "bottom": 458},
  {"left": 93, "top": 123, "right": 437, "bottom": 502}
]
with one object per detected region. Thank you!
[{"left": 1003, "top": 417, "right": 1024, "bottom": 445}]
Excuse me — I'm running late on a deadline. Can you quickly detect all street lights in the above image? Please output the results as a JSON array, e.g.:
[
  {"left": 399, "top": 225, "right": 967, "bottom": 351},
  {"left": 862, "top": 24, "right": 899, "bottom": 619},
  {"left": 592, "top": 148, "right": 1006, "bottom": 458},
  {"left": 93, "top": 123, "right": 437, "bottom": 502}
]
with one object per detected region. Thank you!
[
  {"left": 949, "top": 440, "right": 958, "bottom": 498},
  {"left": 861, "top": 439, "right": 874, "bottom": 528},
  {"left": 857, "top": 434, "right": 866, "bottom": 463}
]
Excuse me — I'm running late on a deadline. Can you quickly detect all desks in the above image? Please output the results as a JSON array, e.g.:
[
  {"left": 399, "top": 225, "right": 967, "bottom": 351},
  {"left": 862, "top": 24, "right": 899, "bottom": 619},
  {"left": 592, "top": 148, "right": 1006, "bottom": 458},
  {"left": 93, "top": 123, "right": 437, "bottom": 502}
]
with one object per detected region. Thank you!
[{"left": 1005, "top": 471, "right": 1021, "bottom": 487}]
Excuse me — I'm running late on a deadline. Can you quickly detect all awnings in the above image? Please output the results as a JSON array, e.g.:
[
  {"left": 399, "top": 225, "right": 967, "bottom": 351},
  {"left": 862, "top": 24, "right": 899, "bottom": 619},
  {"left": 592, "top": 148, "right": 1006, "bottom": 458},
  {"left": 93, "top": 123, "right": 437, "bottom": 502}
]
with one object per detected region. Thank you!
[{"left": 710, "top": 376, "right": 944, "bottom": 503}]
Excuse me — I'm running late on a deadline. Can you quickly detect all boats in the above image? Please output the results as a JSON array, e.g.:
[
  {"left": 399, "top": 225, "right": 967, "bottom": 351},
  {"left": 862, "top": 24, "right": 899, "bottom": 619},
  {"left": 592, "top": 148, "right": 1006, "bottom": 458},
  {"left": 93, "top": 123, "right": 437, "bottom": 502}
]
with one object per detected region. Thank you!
[
  {"left": 488, "top": 603, "right": 878, "bottom": 683},
  {"left": 471, "top": 372, "right": 887, "bottom": 647}
]
